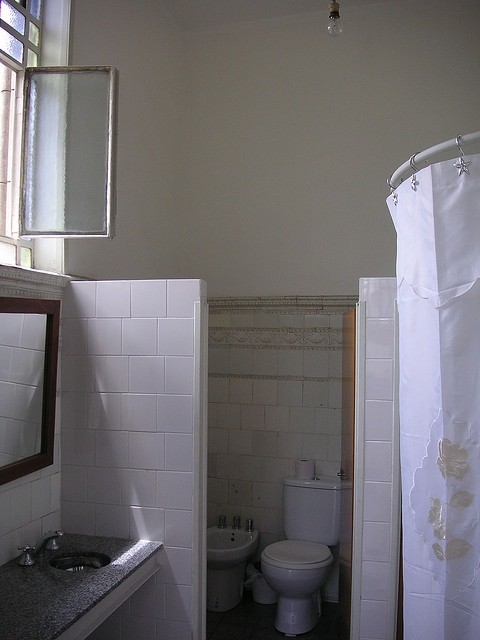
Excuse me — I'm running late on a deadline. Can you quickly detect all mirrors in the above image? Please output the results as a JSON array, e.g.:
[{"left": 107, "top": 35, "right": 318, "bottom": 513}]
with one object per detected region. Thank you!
[{"left": 0, "top": 296, "right": 62, "bottom": 486}]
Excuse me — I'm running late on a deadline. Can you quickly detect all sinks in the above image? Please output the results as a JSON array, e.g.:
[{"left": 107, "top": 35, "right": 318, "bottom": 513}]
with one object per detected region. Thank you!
[
  {"left": 207, "top": 527, "right": 253, "bottom": 550},
  {"left": 50, "top": 551, "right": 111, "bottom": 573}
]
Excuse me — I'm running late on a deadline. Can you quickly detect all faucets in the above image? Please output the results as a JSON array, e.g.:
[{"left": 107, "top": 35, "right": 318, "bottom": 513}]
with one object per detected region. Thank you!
[{"left": 34, "top": 530, "right": 63, "bottom": 558}]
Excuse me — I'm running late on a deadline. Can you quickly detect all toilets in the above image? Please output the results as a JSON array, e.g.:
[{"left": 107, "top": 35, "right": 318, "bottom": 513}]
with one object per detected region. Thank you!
[{"left": 260, "top": 477, "right": 341, "bottom": 635}]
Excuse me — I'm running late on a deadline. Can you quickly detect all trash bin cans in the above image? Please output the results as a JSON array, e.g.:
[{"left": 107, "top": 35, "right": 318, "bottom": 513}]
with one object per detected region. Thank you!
[{"left": 244, "top": 561, "right": 279, "bottom": 604}]
